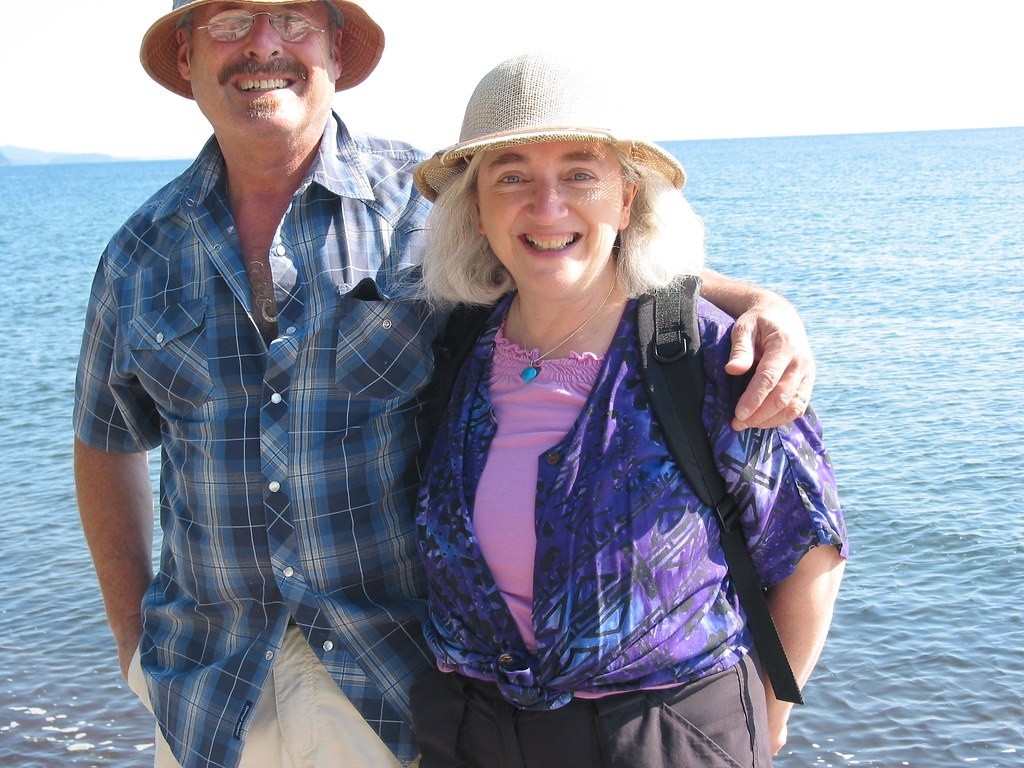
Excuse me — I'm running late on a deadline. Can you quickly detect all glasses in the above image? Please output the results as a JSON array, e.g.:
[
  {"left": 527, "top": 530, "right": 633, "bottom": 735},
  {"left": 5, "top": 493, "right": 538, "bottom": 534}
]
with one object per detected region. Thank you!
[{"left": 188, "top": 9, "right": 332, "bottom": 43}]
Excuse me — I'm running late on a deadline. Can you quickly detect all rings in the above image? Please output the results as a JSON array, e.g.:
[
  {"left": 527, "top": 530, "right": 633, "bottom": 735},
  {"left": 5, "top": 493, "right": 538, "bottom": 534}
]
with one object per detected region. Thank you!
[{"left": 793, "top": 391, "right": 807, "bottom": 403}]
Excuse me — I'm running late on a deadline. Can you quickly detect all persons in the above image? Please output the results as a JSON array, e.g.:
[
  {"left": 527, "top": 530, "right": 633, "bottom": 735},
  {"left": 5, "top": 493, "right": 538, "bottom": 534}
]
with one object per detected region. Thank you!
[
  {"left": 408, "top": 52, "right": 849, "bottom": 768},
  {"left": 71, "top": 0, "right": 816, "bottom": 768}
]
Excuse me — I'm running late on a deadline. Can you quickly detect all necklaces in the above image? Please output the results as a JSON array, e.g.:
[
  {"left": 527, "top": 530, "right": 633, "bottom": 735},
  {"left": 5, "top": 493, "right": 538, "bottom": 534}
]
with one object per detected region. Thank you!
[{"left": 517, "top": 275, "right": 616, "bottom": 384}]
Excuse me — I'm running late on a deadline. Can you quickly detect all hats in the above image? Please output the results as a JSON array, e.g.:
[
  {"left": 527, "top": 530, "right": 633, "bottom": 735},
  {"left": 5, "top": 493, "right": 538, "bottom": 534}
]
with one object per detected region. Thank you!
[
  {"left": 139, "top": 0, "right": 385, "bottom": 102},
  {"left": 413, "top": 56, "right": 688, "bottom": 204}
]
